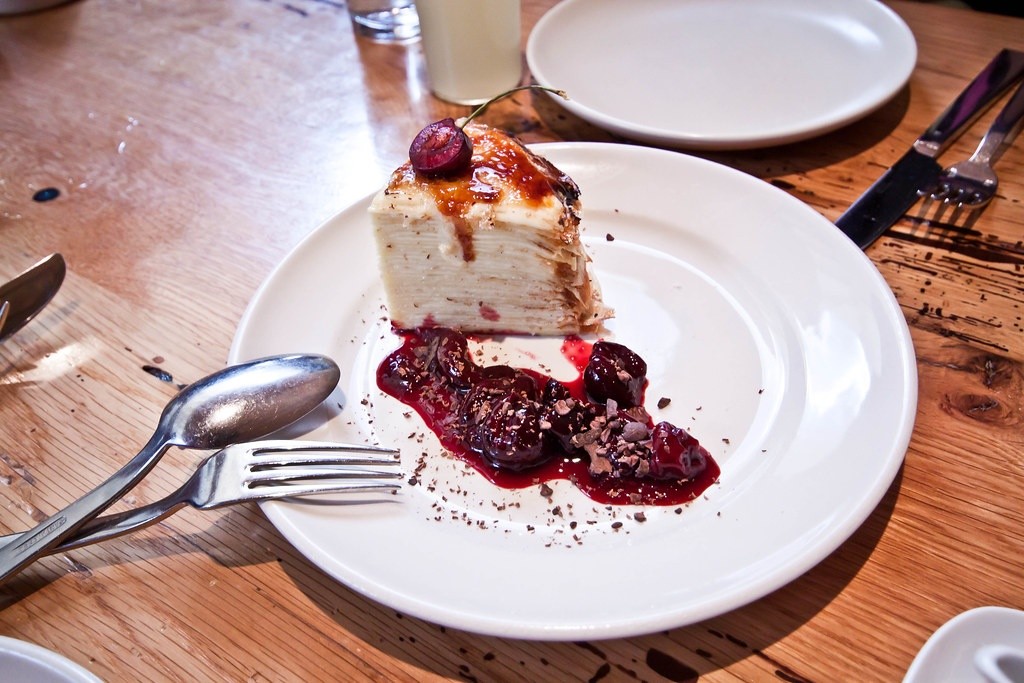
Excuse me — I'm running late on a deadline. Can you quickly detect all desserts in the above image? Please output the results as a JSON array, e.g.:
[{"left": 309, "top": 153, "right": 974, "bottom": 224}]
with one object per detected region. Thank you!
[{"left": 367, "top": 116, "right": 616, "bottom": 337}]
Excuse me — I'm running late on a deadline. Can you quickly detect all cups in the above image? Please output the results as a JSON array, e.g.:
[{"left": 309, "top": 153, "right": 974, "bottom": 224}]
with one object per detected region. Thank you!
[
  {"left": 346, "top": 0, "right": 422, "bottom": 46},
  {"left": 410, "top": 2, "right": 523, "bottom": 104}
]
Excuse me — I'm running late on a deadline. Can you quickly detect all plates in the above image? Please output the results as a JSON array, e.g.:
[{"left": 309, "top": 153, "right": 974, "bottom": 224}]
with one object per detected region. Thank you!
[
  {"left": 517, "top": 0, "right": 918, "bottom": 154},
  {"left": 0, "top": 635, "right": 108, "bottom": 683},
  {"left": 219, "top": 138, "right": 919, "bottom": 643},
  {"left": 900, "top": 605, "right": 1024, "bottom": 683}
]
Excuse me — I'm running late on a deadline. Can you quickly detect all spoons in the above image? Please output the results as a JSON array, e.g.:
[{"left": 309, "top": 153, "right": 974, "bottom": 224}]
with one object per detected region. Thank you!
[
  {"left": 0, "top": 351, "right": 341, "bottom": 589},
  {"left": 0, "top": 249, "right": 66, "bottom": 343}
]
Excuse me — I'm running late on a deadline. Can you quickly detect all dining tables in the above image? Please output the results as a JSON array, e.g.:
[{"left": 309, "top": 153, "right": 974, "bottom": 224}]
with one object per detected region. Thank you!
[{"left": 0, "top": 0, "right": 1024, "bottom": 683}]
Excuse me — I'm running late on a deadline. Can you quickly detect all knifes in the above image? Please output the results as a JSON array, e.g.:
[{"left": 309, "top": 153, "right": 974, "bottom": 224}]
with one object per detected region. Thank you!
[{"left": 831, "top": 49, "right": 1024, "bottom": 252}]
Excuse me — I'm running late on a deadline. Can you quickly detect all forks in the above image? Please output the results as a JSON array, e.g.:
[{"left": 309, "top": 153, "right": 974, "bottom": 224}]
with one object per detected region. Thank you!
[
  {"left": 915, "top": 80, "right": 1024, "bottom": 210},
  {"left": 0, "top": 436, "right": 405, "bottom": 561}
]
